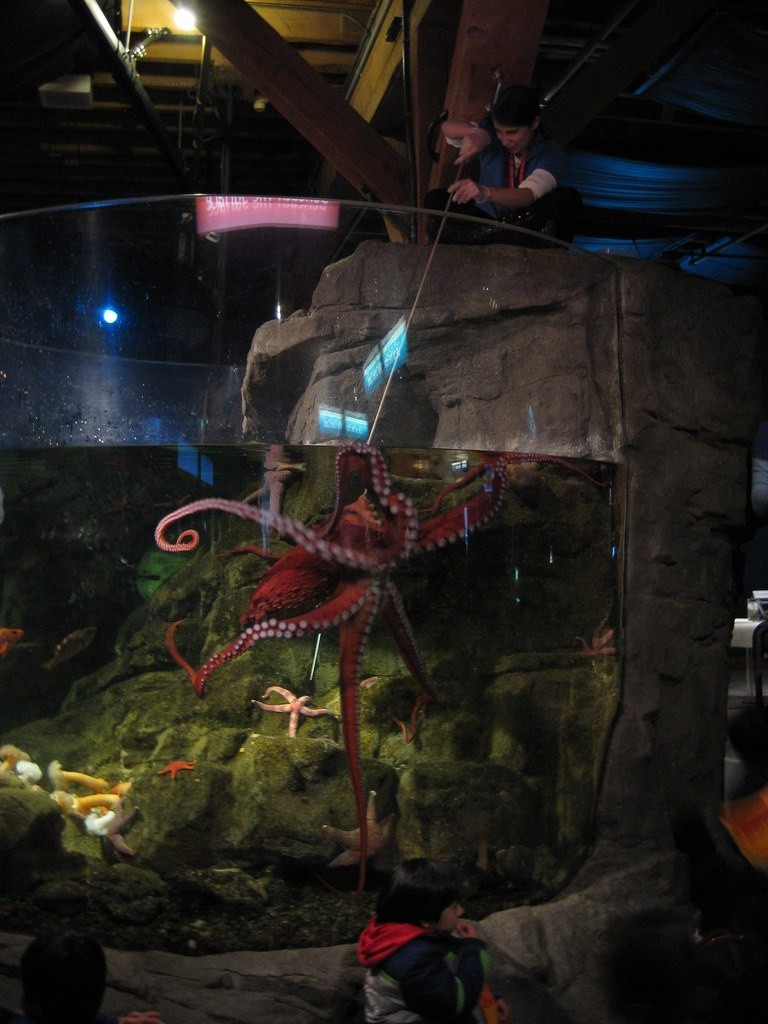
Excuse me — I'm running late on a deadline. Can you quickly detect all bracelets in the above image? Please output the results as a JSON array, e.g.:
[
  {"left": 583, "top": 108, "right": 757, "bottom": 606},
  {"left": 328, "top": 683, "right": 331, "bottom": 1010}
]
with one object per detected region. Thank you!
[
  {"left": 474, "top": 186, "right": 491, "bottom": 205},
  {"left": 472, "top": 127, "right": 492, "bottom": 145}
]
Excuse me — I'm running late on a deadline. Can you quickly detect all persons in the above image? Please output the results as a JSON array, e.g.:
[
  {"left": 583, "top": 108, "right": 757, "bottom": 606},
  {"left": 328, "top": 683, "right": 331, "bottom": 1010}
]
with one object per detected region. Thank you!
[
  {"left": 424, "top": 84, "right": 584, "bottom": 249},
  {"left": 20, "top": 928, "right": 164, "bottom": 1024},
  {"left": 364, "top": 856, "right": 510, "bottom": 1024}
]
